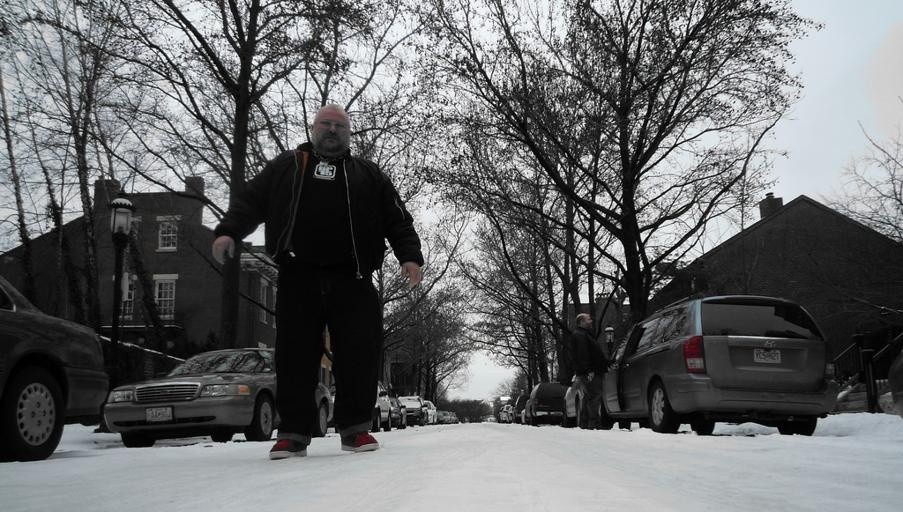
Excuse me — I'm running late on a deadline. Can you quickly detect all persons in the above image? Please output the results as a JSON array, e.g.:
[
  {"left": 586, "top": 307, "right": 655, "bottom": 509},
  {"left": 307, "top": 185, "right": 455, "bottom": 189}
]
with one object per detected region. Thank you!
[
  {"left": 209, "top": 104, "right": 425, "bottom": 459},
  {"left": 567, "top": 313, "right": 611, "bottom": 430}
]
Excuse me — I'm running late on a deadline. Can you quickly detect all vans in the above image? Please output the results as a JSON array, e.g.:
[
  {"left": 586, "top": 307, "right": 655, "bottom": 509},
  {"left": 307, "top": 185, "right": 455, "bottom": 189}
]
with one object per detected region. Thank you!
[{"left": 598, "top": 292, "right": 837, "bottom": 436}]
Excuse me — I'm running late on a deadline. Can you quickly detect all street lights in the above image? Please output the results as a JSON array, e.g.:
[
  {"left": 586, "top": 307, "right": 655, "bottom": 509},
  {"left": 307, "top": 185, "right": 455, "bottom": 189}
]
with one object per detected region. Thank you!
[
  {"left": 94, "top": 197, "right": 135, "bottom": 433},
  {"left": 605, "top": 324, "right": 615, "bottom": 359}
]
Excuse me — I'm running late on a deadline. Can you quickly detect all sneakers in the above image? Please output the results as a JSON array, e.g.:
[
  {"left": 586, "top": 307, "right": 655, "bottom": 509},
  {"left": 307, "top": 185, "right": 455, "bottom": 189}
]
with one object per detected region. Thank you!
[
  {"left": 269, "top": 439, "right": 309, "bottom": 460},
  {"left": 340, "top": 433, "right": 379, "bottom": 452}
]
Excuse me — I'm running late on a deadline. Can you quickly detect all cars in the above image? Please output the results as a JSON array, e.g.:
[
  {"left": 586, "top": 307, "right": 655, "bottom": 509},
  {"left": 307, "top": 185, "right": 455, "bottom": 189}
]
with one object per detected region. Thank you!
[
  {"left": 498, "top": 374, "right": 585, "bottom": 426},
  {"left": 103, "top": 348, "right": 333, "bottom": 448},
  {"left": 327, "top": 380, "right": 459, "bottom": 433},
  {"left": 0, "top": 274, "right": 110, "bottom": 457}
]
